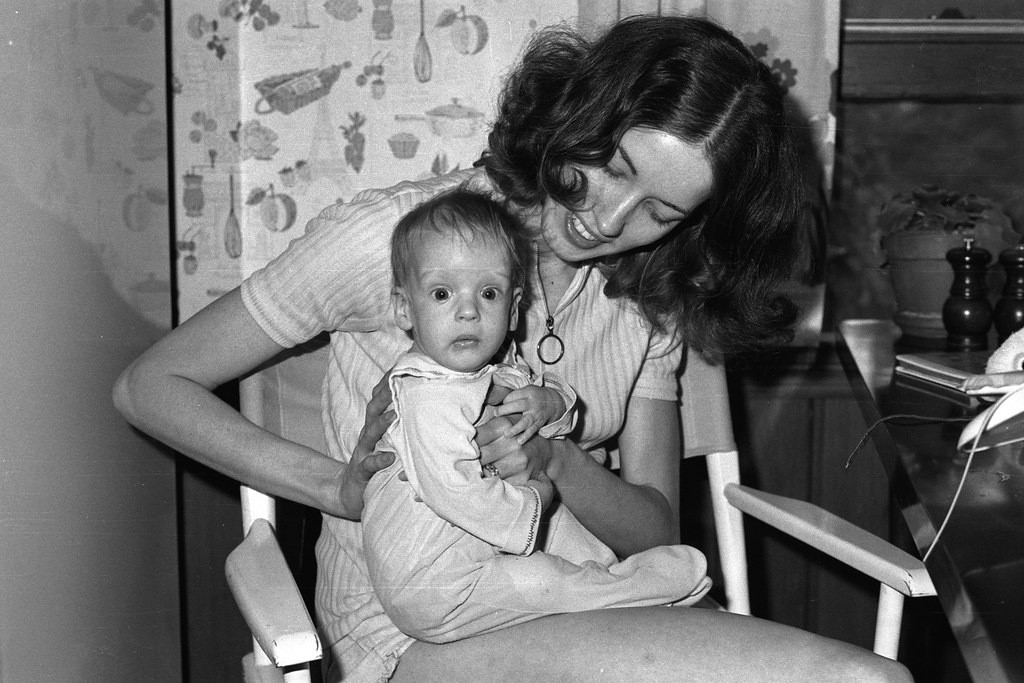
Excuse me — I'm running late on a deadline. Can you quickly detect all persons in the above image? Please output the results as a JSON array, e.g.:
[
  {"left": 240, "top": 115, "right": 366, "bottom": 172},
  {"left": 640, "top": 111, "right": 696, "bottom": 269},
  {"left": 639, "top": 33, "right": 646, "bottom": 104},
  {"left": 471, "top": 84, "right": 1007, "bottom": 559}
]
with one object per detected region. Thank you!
[
  {"left": 108, "top": 15, "right": 917, "bottom": 683},
  {"left": 358, "top": 189, "right": 712, "bottom": 645}
]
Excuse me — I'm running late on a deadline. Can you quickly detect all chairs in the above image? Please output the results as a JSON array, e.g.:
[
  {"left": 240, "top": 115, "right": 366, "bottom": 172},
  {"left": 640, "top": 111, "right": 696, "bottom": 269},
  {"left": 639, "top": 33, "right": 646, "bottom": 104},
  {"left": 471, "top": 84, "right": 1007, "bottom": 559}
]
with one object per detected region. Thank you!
[{"left": 225, "top": 316, "right": 943, "bottom": 683}]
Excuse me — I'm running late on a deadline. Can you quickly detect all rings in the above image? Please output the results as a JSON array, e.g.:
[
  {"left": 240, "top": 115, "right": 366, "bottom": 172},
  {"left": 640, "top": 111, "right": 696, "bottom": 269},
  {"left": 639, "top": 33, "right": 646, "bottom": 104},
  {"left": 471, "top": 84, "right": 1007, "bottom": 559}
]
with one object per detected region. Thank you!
[{"left": 485, "top": 463, "right": 502, "bottom": 479}]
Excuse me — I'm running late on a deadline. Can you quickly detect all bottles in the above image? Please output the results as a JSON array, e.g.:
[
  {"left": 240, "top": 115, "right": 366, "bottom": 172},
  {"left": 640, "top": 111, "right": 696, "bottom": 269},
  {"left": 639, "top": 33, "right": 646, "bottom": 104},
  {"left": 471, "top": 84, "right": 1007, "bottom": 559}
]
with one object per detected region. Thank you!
[
  {"left": 940, "top": 237, "right": 993, "bottom": 349},
  {"left": 992, "top": 241, "right": 1023, "bottom": 347}
]
